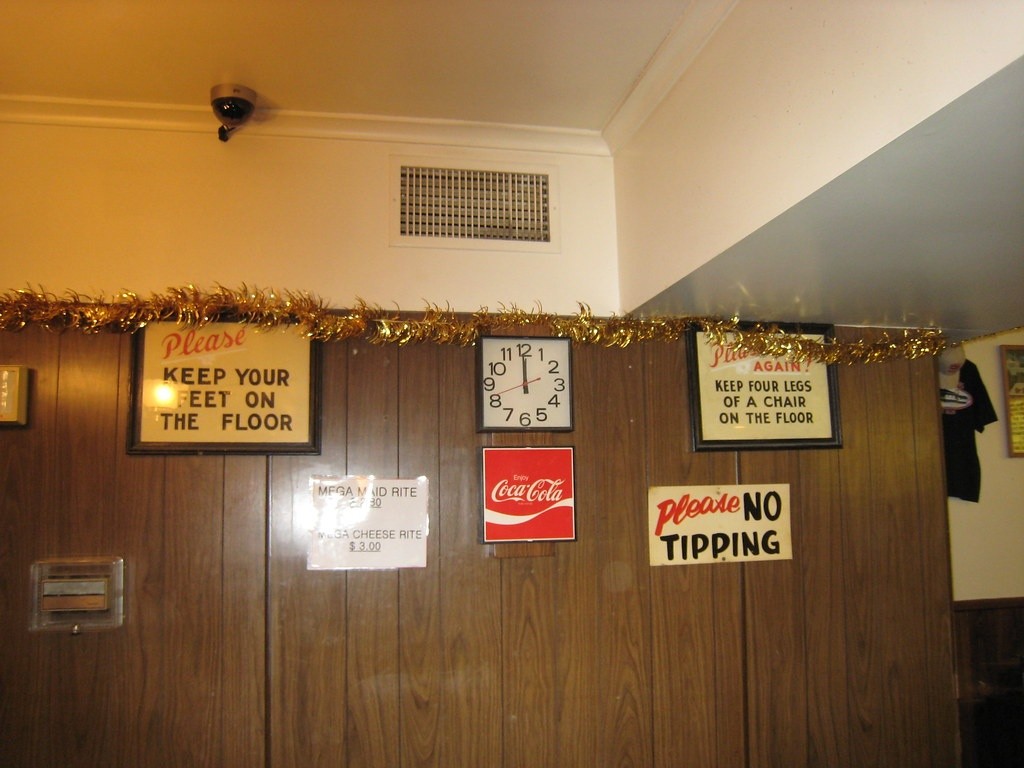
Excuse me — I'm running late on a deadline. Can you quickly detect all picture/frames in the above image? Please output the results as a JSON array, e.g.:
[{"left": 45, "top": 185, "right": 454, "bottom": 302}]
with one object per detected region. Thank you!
[
  {"left": 685, "top": 322, "right": 843, "bottom": 451},
  {"left": 999, "top": 344, "right": 1024, "bottom": 458},
  {"left": 127, "top": 314, "right": 323, "bottom": 456}
]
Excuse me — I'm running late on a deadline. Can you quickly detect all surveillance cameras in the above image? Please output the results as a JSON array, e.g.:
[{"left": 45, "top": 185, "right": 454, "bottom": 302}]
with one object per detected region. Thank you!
[{"left": 209, "top": 84, "right": 258, "bottom": 128}]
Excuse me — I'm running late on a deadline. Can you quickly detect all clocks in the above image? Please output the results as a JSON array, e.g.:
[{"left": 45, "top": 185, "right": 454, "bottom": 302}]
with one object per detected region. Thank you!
[{"left": 473, "top": 335, "right": 575, "bottom": 433}]
